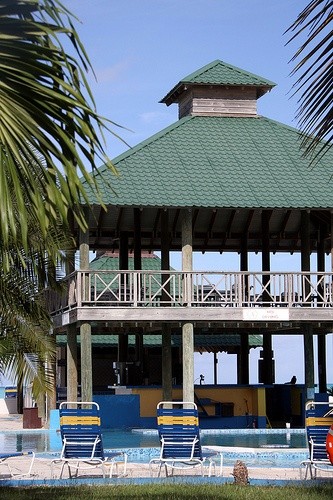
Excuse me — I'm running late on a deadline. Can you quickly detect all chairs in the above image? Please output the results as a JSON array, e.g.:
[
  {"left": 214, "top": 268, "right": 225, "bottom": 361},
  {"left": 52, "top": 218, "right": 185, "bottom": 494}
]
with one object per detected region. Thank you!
[
  {"left": 0, "top": 451, "right": 35, "bottom": 479},
  {"left": 148, "top": 400, "right": 223, "bottom": 478},
  {"left": 299, "top": 401, "right": 332, "bottom": 482},
  {"left": 49, "top": 401, "right": 128, "bottom": 479}
]
